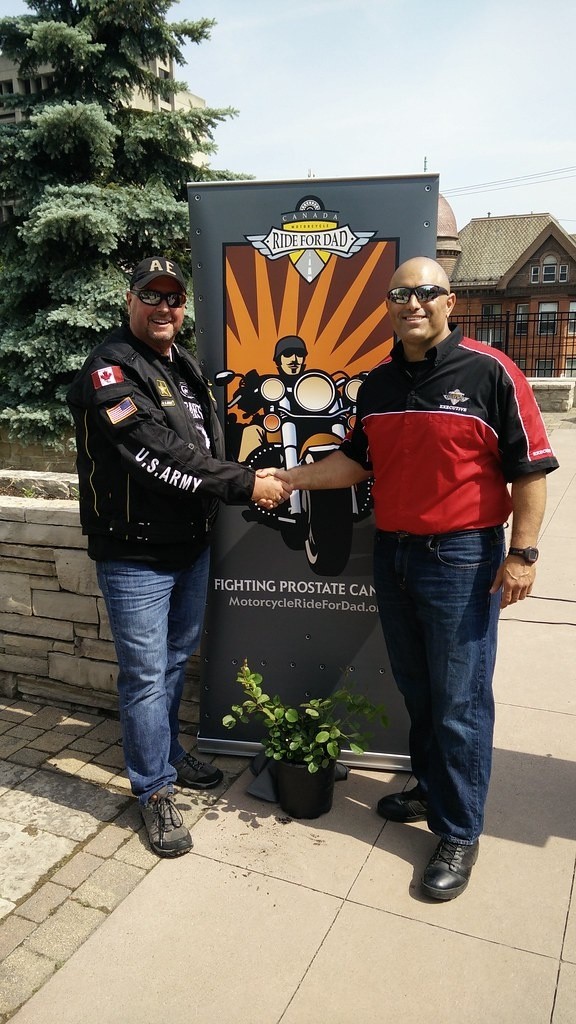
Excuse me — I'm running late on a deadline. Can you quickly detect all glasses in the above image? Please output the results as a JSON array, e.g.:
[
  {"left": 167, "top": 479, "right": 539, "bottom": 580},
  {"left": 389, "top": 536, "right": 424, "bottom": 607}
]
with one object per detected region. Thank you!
[
  {"left": 130, "top": 289, "right": 187, "bottom": 308},
  {"left": 387, "top": 284, "right": 450, "bottom": 304}
]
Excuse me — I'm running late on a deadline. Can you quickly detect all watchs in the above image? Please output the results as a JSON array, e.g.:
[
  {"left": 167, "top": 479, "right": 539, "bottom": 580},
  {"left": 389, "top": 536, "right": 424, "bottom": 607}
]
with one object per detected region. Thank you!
[{"left": 508, "top": 545, "right": 538, "bottom": 562}]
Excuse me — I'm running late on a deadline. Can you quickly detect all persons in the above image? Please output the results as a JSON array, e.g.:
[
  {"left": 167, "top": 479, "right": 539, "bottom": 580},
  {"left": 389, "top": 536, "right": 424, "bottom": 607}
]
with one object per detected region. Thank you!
[
  {"left": 262, "top": 257, "right": 560, "bottom": 899},
  {"left": 65, "top": 258, "right": 292, "bottom": 859}
]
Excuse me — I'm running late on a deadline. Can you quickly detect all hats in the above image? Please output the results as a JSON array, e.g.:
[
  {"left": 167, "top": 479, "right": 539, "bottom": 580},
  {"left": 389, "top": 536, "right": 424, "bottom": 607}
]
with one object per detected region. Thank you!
[{"left": 130, "top": 257, "right": 187, "bottom": 296}]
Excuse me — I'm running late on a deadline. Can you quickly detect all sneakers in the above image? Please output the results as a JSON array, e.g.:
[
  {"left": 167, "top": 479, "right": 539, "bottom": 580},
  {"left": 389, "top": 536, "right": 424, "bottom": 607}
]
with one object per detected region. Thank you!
[
  {"left": 140, "top": 784, "right": 194, "bottom": 858},
  {"left": 177, "top": 754, "right": 224, "bottom": 790}
]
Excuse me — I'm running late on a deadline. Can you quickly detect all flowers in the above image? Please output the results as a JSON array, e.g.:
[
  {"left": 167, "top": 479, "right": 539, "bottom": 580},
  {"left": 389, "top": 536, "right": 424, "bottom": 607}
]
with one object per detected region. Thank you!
[{"left": 221, "top": 653, "right": 388, "bottom": 782}]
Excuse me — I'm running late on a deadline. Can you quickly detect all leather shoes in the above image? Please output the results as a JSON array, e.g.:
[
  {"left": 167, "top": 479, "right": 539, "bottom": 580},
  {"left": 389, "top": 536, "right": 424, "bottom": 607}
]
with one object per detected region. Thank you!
[
  {"left": 421, "top": 840, "right": 479, "bottom": 900},
  {"left": 377, "top": 790, "right": 430, "bottom": 823}
]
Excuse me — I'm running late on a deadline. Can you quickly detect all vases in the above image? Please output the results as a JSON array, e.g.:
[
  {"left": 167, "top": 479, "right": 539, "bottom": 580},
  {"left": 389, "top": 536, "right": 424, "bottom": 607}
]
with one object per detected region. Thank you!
[{"left": 271, "top": 756, "right": 342, "bottom": 820}]
